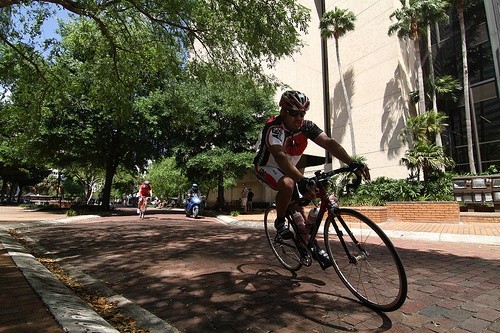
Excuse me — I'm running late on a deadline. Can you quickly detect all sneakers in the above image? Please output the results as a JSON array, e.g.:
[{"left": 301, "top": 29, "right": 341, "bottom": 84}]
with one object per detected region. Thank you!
[
  {"left": 274, "top": 219, "right": 294, "bottom": 240},
  {"left": 310, "top": 248, "right": 332, "bottom": 263}
]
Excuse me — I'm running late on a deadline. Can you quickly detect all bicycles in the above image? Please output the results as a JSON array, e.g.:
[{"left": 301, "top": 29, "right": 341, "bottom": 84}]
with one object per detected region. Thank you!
[
  {"left": 264, "top": 166, "right": 410, "bottom": 312},
  {"left": 139, "top": 197, "right": 147, "bottom": 219}
]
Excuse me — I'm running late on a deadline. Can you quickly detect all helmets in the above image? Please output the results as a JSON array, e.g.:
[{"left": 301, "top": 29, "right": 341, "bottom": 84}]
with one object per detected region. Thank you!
[
  {"left": 144, "top": 181, "right": 150, "bottom": 185},
  {"left": 192, "top": 184, "right": 198, "bottom": 190},
  {"left": 279, "top": 90, "right": 310, "bottom": 110}
]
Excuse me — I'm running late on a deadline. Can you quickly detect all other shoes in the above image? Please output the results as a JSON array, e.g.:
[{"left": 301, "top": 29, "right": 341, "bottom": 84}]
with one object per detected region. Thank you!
[{"left": 137, "top": 209, "right": 140, "bottom": 213}]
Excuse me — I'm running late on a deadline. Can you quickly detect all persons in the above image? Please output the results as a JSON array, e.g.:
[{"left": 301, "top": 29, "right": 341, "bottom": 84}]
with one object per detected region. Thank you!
[
  {"left": 137, "top": 181, "right": 153, "bottom": 213},
  {"left": 122, "top": 194, "right": 176, "bottom": 209},
  {"left": 255, "top": 89, "right": 370, "bottom": 268},
  {"left": 186, "top": 184, "right": 201, "bottom": 208},
  {"left": 248, "top": 188, "right": 254, "bottom": 210},
  {"left": 241, "top": 184, "right": 249, "bottom": 213}
]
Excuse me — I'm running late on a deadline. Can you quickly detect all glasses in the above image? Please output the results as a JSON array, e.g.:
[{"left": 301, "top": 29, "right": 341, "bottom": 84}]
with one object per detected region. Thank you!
[{"left": 285, "top": 109, "right": 305, "bottom": 118}]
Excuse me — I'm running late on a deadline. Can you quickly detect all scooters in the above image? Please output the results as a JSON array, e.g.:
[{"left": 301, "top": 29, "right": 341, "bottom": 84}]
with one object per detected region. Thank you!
[{"left": 184, "top": 194, "right": 205, "bottom": 219}]
